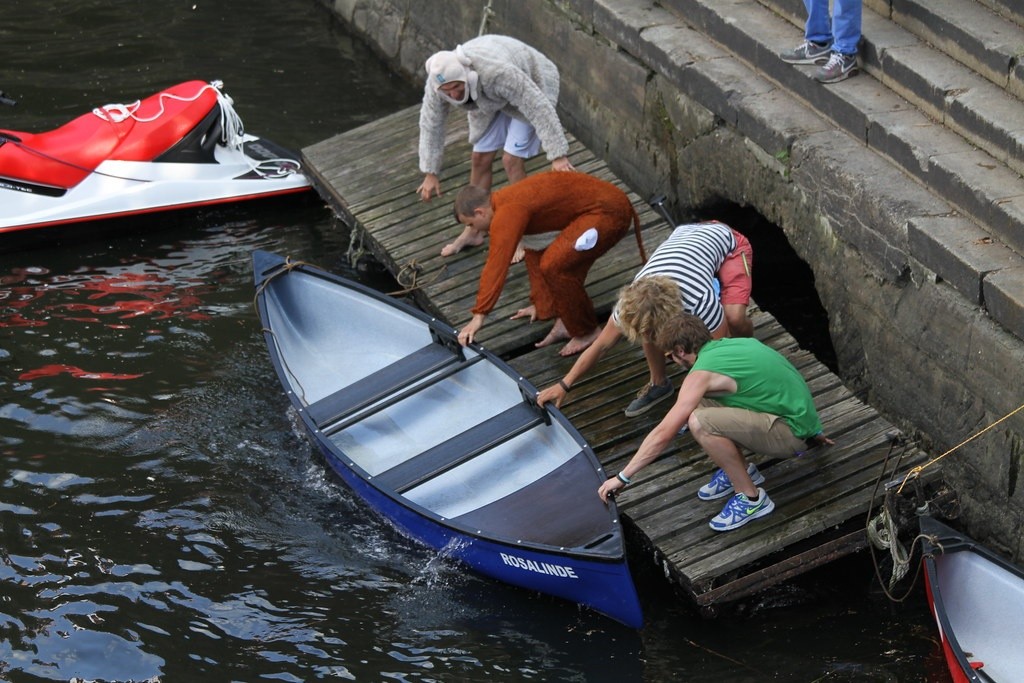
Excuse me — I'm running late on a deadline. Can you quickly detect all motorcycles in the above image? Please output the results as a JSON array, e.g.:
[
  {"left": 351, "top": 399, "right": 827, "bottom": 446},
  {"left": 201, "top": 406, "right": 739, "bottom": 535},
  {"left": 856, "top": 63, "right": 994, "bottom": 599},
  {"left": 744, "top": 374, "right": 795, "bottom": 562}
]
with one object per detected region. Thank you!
[{"left": 0, "top": 80, "right": 312, "bottom": 254}]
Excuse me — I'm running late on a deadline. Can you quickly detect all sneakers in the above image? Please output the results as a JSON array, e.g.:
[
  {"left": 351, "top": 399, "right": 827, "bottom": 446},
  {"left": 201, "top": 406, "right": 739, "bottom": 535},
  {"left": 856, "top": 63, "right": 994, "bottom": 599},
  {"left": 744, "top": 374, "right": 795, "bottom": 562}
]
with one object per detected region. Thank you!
[
  {"left": 697, "top": 461, "right": 766, "bottom": 501},
  {"left": 624, "top": 374, "right": 674, "bottom": 417},
  {"left": 780, "top": 37, "right": 834, "bottom": 65},
  {"left": 709, "top": 486, "right": 774, "bottom": 531},
  {"left": 814, "top": 51, "right": 859, "bottom": 83}
]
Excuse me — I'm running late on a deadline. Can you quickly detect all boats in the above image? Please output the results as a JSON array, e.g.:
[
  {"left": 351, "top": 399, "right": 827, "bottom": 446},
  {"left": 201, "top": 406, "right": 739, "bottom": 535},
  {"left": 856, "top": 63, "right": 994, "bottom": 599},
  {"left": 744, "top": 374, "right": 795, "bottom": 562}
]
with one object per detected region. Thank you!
[
  {"left": 920, "top": 515, "right": 1024, "bottom": 683},
  {"left": 251, "top": 249, "right": 644, "bottom": 631}
]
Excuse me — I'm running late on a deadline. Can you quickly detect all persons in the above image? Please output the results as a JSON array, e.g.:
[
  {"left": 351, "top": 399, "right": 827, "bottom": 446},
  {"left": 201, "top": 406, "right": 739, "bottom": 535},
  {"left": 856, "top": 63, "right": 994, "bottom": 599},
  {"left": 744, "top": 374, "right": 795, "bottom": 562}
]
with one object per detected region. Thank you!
[
  {"left": 536, "top": 220, "right": 752, "bottom": 416},
  {"left": 452, "top": 170, "right": 647, "bottom": 357},
  {"left": 598, "top": 311, "right": 835, "bottom": 531},
  {"left": 417, "top": 34, "right": 577, "bottom": 263},
  {"left": 779, "top": 0, "right": 861, "bottom": 83}
]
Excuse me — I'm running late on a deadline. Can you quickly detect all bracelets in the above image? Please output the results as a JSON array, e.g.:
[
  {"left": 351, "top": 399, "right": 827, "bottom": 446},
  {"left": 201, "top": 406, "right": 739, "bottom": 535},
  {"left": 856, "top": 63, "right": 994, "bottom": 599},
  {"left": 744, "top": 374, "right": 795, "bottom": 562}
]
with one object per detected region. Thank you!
[
  {"left": 558, "top": 379, "right": 569, "bottom": 392},
  {"left": 614, "top": 471, "right": 631, "bottom": 485}
]
